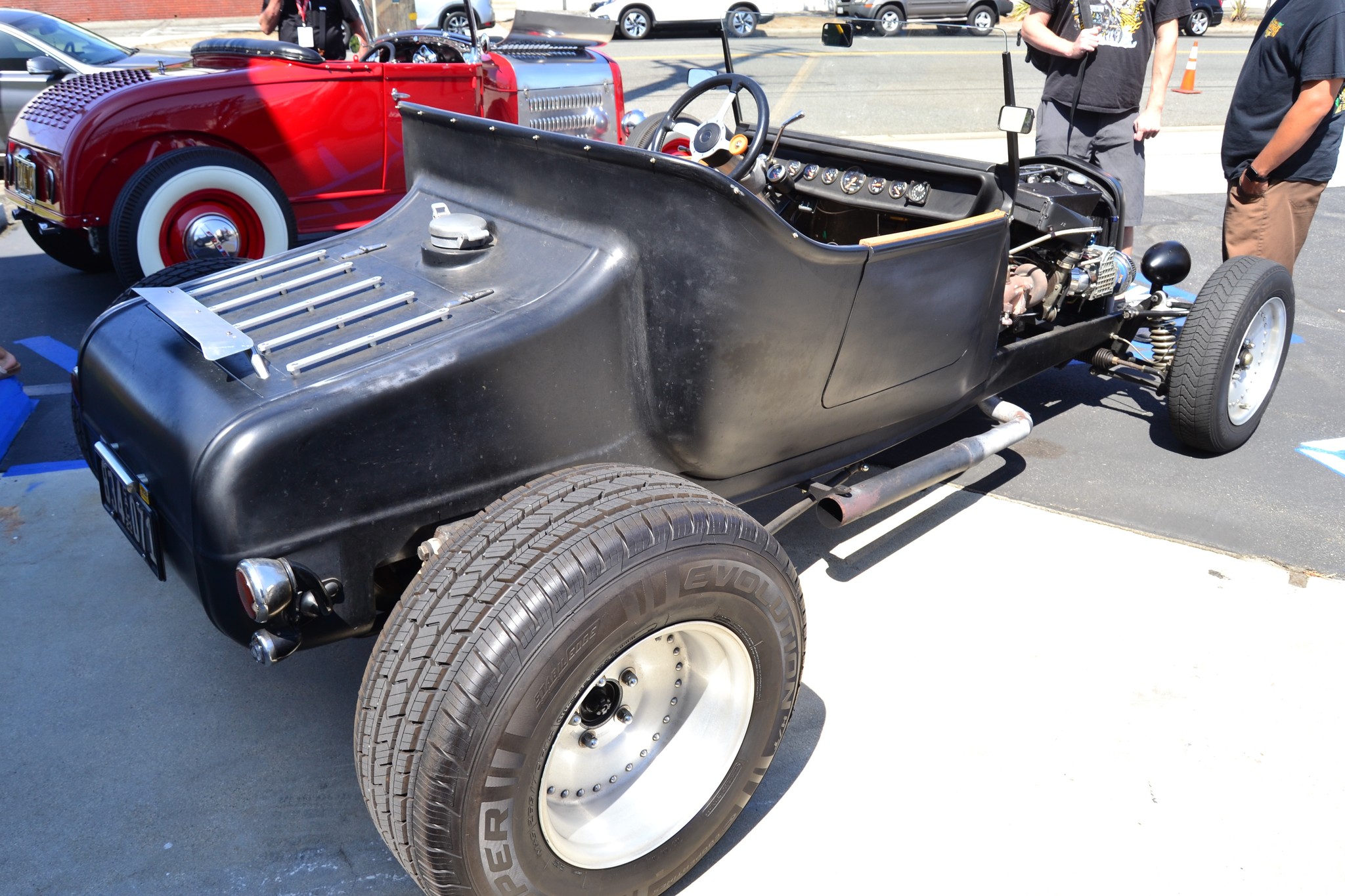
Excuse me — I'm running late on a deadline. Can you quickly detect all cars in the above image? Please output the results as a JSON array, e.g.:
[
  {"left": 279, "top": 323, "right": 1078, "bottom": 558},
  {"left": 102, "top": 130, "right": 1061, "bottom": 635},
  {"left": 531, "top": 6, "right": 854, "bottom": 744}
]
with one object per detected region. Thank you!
[
  {"left": 1176, "top": 1, "right": 1223, "bottom": 36},
  {"left": 69, "top": 16, "right": 1296, "bottom": 896},
  {"left": 589, "top": 1, "right": 805, "bottom": 38},
  {"left": 1, "top": 8, "right": 193, "bottom": 181},
  {"left": 834, "top": 0, "right": 1014, "bottom": 37},
  {"left": 2, "top": 27, "right": 704, "bottom": 292},
  {"left": 415, "top": 2, "right": 497, "bottom": 34}
]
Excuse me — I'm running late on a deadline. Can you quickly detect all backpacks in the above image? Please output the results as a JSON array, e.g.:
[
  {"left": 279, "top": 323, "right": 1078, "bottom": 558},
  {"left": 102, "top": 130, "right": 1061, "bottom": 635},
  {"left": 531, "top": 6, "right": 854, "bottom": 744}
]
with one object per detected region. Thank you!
[{"left": 1016, "top": 0, "right": 1064, "bottom": 76}]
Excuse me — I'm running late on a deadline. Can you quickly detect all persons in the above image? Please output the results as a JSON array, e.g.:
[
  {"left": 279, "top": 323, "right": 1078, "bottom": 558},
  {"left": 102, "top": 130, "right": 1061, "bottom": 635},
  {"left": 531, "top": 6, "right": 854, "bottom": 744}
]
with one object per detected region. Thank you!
[
  {"left": 1211, "top": 0, "right": 1345, "bottom": 281},
  {"left": 258, "top": 0, "right": 371, "bottom": 63},
  {"left": 1020, "top": 1, "right": 1194, "bottom": 288}
]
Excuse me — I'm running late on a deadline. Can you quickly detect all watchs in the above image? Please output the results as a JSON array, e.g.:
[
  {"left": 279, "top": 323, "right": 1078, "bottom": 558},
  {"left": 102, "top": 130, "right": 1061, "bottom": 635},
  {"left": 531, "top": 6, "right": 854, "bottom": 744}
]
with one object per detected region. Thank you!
[{"left": 1244, "top": 165, "right": 1271, "bottom": 183}]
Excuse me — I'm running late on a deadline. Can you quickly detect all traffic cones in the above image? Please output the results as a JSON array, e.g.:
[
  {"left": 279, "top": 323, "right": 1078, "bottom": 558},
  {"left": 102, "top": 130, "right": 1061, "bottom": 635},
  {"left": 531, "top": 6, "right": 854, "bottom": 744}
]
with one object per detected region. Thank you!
[{"left": 1169, "top": 41, "right": 1203, "bottom": 94}]
